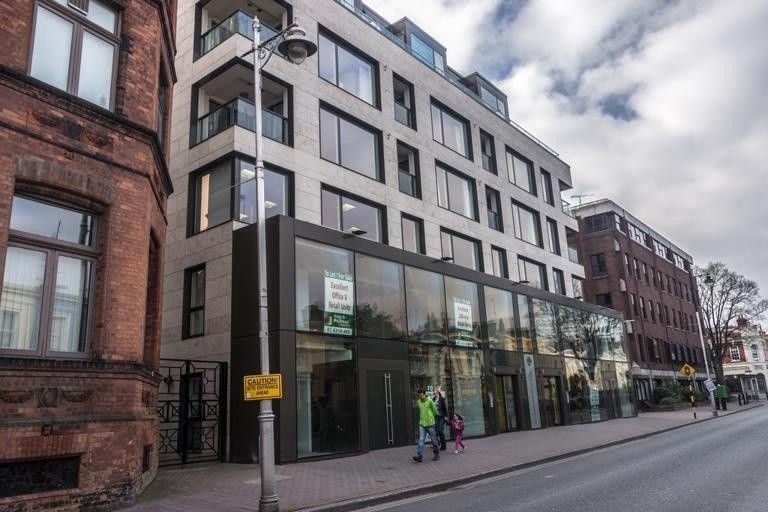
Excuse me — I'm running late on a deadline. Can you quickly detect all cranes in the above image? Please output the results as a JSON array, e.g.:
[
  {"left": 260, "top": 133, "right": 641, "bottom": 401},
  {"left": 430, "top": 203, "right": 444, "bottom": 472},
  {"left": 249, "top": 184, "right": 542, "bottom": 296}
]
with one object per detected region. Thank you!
[{"left": 570, "top": 193, "right": 595, "bottom": 205}]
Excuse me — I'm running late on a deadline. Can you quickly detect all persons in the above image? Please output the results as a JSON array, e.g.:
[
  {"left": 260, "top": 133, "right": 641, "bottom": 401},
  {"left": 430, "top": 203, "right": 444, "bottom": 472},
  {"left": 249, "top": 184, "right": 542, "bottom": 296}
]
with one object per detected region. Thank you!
[
  {"left": 411, "top": 387, "right": 442, "bottom": 463},
  {"left": 447, "top": 411, "right": 466, "bottom": 455},
  {"left": 432, "top": 386, "right": 449, "bottom": 455}
]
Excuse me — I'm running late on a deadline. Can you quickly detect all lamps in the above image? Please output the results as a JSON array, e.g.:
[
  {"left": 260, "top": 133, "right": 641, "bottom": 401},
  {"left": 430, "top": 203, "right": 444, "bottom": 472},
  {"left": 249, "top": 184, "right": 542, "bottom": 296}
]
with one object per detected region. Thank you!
[
  {"left": 516, "top": 280, "right": 530, "bottom": 284},
  {"left": 573, "top": 296, "right": 583, "bottom": 300},
  {"left": 352, "top": 226, "right": 367, "bottom": 235},
  {"left": 440, "top": 257, "right": 453, "bottom": 263}
]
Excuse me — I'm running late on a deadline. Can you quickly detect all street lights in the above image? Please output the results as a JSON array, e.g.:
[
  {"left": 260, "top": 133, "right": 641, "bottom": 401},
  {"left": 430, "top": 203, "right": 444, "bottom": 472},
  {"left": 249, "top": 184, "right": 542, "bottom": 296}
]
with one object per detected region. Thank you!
[
  {"left": 689, "top": 269, "right": 718, "bottom": 416},
  {"left": 240, "top": 14, "right": 317, "bottom": 512}
]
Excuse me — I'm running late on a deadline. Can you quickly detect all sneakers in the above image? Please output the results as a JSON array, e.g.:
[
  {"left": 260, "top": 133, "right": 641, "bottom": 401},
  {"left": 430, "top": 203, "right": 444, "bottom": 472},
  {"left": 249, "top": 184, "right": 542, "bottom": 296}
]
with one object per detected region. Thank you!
[
  {"left": 430, "top": 446, "right": 447, "bottom": 451},
  {"left": 454, "top": 445, "right": 466, "bottom": 454},
  {"left": 432, "top": 454, "right": 440, "bottom": 461},
  {"left": 413, "top": 456, "right": 423, "bottom": 463}
]
